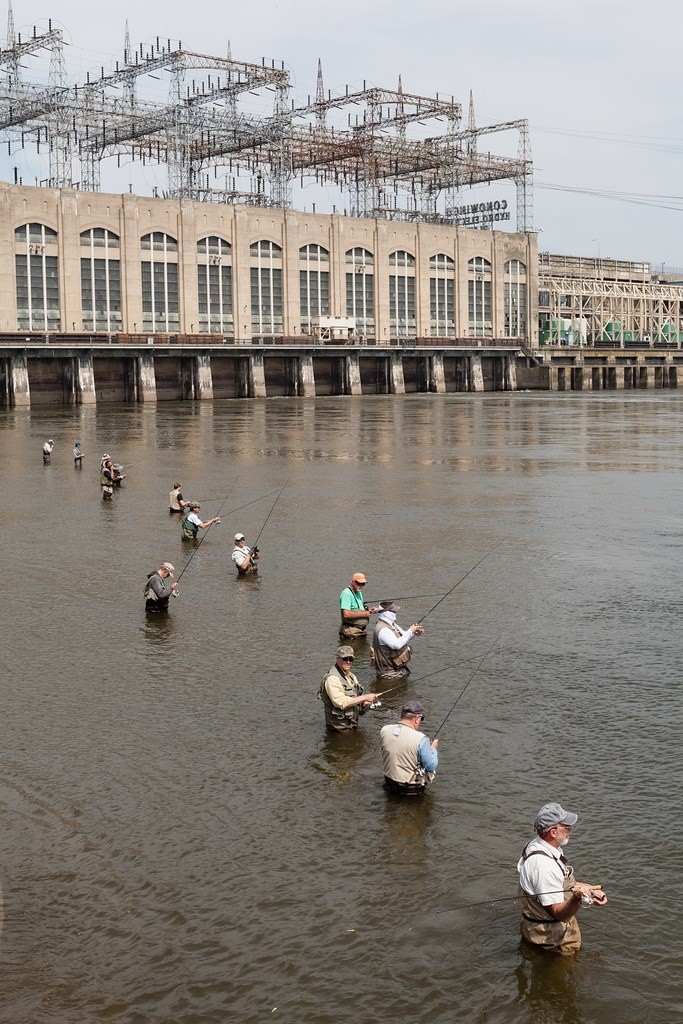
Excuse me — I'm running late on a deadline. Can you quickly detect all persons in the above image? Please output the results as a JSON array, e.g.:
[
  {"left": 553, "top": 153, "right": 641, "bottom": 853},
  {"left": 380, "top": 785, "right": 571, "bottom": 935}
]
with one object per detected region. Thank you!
[
  {"left": 380, "top": 700, "right": 439, "bottom": 797},
  {"left": 170, "top": 482, "right": 190, "bottom": 513},
  {"left": 43, "top": 439, "right": 54, "bottom": 463},
  {"left": 371, "top": 601, "right": 424, "bottom": 679},
  {"left": 232, "top": 533, "right": 260, "bottom": 575},
  {"left": 143, "top": 562, "right": 178, "bottom": 613},
  {"left": 338, "top": 573, "right": 378, "bottom": 640},
  {"left": 73, "top": 442, "right": 85, "bottom": 466},
  {"left": 100, "top": 454, "right": 126, "bottom": 500},
  {"left": 517, "top": 803, "right": 607, "bottom": 956},
  {"left": 317, "top": 646, "right": 378, "bottom": 733},
  {"left": 181, "top": 501, "right": 220, "bottom": 542}
]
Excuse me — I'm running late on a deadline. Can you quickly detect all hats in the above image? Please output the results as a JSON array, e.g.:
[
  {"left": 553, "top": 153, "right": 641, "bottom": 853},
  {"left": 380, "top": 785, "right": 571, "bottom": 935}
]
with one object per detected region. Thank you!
[
  {"left": 533, "top": 803, "right": 578, "bottom": 832},
  {"left": 190, "top": 501, "right": 202, "bottom": 507},
  {"left": 335, "top": 646, "right": 355, "bottom": 658},
  {"left": 163, "top": 563, "right": 175, "bottom": 577},
  {"left": 75, "top": 441, "right": 81, "bottom": 446},
  {"left": 101, "top": 454, "right": 123, "bottom": 470},
  {"left": 378, "top": 603, "right": 401, "bottom": 613},
  {"left": 234, "top": 533, "right": 244, "bottom": 540},
  {"left": 352, "top": 573, "right": 368, "bottom": 584},
  {"left": 48, "top": 439, "right": 54, "bottom": 443},
  {"left": 402, "top": 700, "right": 426, "bottom": 717}
]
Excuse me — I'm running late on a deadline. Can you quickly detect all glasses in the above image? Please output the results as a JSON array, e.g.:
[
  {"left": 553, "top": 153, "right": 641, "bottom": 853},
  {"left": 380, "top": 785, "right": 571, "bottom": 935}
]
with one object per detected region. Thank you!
[
  {"left": 235, "top": 538, "right": 245, "bottom": 541},
  {"left": 418, "top": 715, "right": 424, "bottom": 721},
  {"left": 341, "top": 657, "right": 354, "bottom": 662},
  {"left": 355, "top": 581, "right": 365, "bottom": 586}
]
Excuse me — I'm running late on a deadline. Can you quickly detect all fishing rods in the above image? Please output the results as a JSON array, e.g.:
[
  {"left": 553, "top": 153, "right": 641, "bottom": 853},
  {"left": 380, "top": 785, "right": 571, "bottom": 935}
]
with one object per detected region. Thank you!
[
  {"left": 121, "top": 452, "right": 152, "bottom": 473},
  {"left": 253, "top": 468, "right": 294, "bottom": 547},
  {"left": 369, "top": 641, "right": 515, "bottom": 710},
  {"left": 415, "top": 532, "right": 511, "bottom": 637},
  {"left": 364, "top": 590, "right": 465, "bottom": 611},
  {"left": 55, "top": 421, "right": 73, "bottom": 443},
  {"left": 171, "top": 475, "right": 239, "bottom": 598},
  {"left": 215, "top": 478, "right": 304, "bottom": 526},
  {"left": 197, "top": 497, "right": 232, "bottom": 502},
  {"left": 435, "top": 885, "right": 604, "bottom": 915},
  {"left": 85, "top": 448, "right": 105, "bottom": 455},
  {"left": 433, "top": 637, "right": 501, "bottom": 740}
]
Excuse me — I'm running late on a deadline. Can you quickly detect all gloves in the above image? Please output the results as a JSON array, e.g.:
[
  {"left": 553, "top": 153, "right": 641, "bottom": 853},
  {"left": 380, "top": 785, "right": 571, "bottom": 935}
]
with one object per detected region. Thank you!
[{"left": 247, "top": 546, "right": 260, "bottom": 557}]
